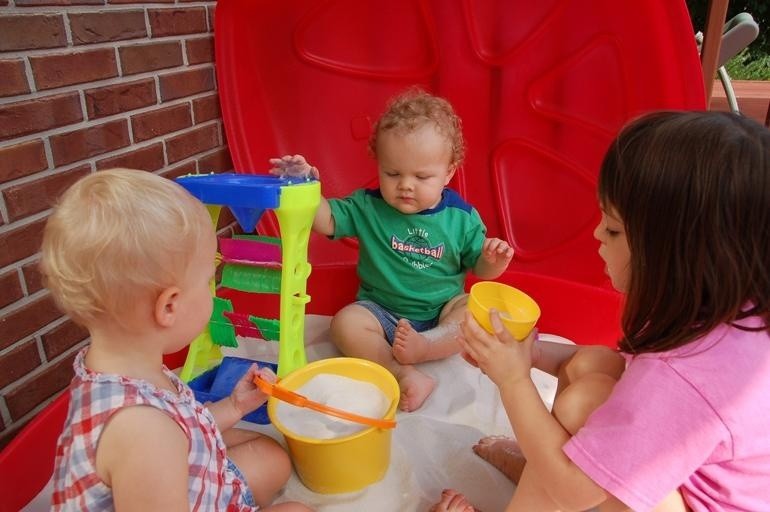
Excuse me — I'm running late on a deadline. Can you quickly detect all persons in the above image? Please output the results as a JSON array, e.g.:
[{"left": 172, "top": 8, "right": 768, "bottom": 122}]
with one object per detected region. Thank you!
[
  {"left": 39, "top": 166, "right": 478, "bottom": 511},
  {"left": 263, "top": 86, "right": 515, "bottom": 414},
  {"left": 449, "top": 105, "right": 767, "bottom": 511}
]
